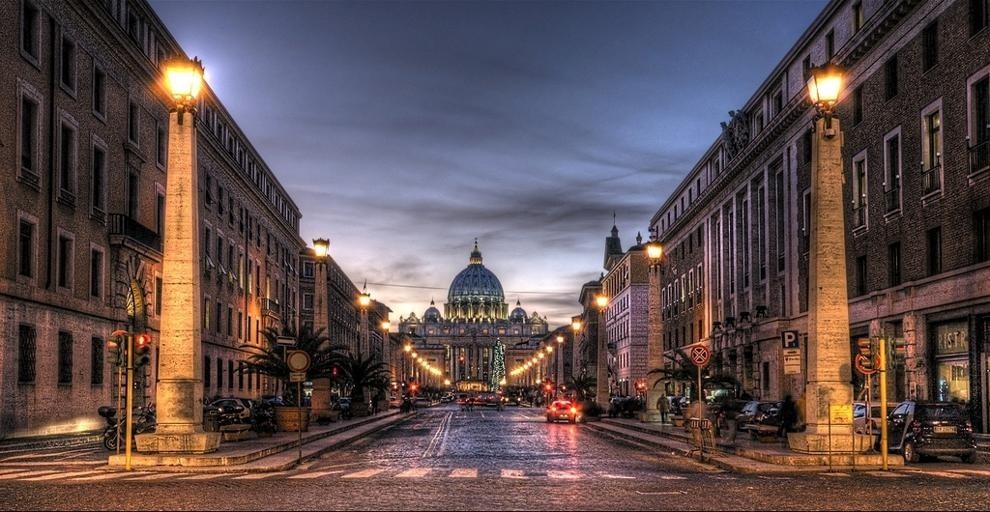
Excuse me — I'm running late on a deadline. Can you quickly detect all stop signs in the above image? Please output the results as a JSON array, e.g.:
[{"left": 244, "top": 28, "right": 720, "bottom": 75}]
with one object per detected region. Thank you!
[{"left": 286, "top": 350, "right": 311, "bottom": 373}]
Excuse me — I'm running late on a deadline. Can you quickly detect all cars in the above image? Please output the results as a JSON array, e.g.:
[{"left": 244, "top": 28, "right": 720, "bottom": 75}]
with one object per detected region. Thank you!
[
  {"left": 414, "top": 391, "right": 517, "bottom": 412},
  {"left": 203, "top": 393, "right": 352, "bottom": 431},
  {"left": 546, "top": 400, "right": 577, "bottom": 424},
  {"left": 666, "top": 396, "right": 783, "bottom": 430},
  {"left": 853, "top": 398, "right": 978, "bottom": 465}
]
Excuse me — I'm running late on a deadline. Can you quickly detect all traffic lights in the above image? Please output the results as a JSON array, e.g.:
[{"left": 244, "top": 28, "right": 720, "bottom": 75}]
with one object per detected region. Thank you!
[{"left": 133, "top": 333, "right": 152, "bottom": 369}]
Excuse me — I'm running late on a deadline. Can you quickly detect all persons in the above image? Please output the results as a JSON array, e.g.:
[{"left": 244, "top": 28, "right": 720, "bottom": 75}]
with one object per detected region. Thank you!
[
  {"left": 371, "top": 395, "right": 379, "bottom": 415},
  {"left": 776, "top": 394, "right": 800, "bottom": 448},
  {"left": 656, "top": 392, "right": 671, "bottom": 424}
]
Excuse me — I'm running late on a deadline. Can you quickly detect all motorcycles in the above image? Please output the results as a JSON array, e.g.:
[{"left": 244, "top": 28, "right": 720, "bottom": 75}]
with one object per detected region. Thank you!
[{"left": 98, "top": 400, "right": 156, "bottom": 451}]
